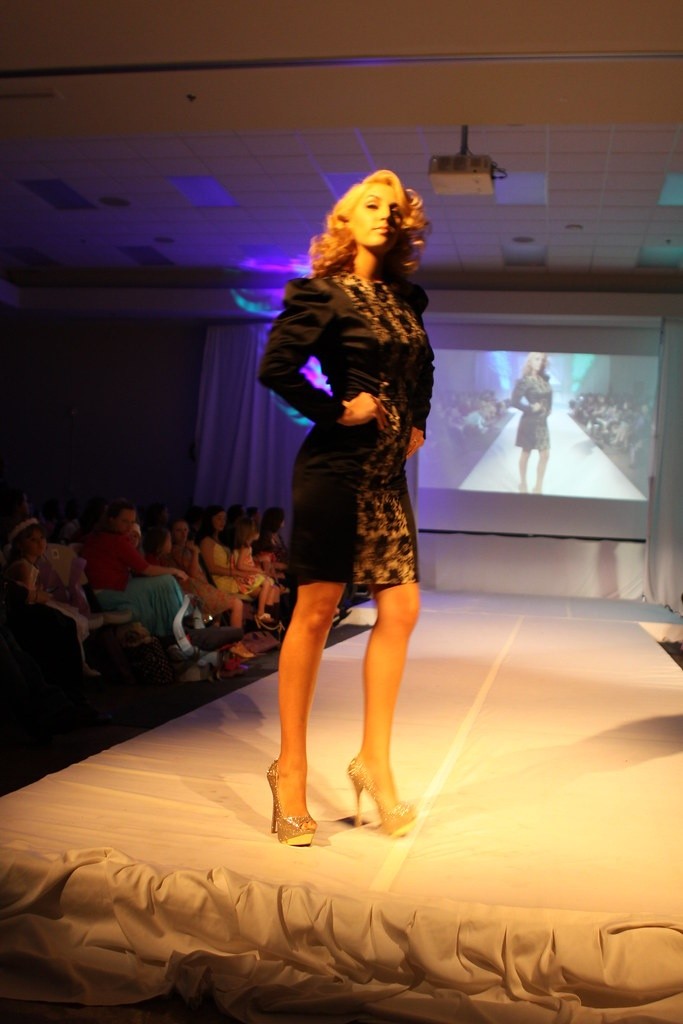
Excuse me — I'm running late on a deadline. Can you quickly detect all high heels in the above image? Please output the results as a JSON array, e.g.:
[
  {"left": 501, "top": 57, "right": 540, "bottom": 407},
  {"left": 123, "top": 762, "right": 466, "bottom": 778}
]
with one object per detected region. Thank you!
[
  {"left": 266, "top": 760, "right": 317, "bottom": 847},
  {"left": 255, "top": 613, "right": 281, "bottom": 630},
  {"left": 349, "top": 756, "right": 418, "bottom": 837}
]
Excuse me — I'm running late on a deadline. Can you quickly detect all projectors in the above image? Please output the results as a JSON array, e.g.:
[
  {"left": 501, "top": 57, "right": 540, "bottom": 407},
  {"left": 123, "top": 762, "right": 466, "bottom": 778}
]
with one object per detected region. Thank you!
[{"left": 429, "top": 155, "right": 495, "bottom": 196}]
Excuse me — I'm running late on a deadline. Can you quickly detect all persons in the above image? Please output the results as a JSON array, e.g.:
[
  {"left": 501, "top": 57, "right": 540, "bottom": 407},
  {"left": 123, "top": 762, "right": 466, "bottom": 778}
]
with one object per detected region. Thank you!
[
  {"left": 568, "top": 381, "right": 656, "bottom": 470},
  {"left": 256, "top": 168, "right": 437, "bottom": 845},
  {"left": 510, "top": 351, "right": 554, "bottom": 495},
  {"left": 0, "top": 488, "right": 376, "bottom": 750},
  {"left": 428, "top": 385, "right": 512, "bottom": 451}
]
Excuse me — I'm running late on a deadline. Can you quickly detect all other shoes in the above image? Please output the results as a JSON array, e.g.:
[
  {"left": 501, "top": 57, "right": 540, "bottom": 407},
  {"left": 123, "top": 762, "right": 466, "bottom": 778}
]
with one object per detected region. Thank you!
[{"left": 83, "top": 668, "right": 102, "bottom": 677}]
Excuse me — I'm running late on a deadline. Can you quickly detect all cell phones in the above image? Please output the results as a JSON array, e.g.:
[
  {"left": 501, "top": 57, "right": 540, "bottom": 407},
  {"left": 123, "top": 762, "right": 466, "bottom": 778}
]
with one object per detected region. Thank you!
[{"left": 43, "top": 586, "right": 58, "bottom": 594}]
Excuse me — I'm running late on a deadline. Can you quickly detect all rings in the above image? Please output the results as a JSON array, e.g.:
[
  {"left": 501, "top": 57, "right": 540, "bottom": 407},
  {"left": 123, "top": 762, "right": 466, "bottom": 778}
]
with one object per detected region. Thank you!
[{"left": 412, "top": 437, "right": 418, "bottom": 444}]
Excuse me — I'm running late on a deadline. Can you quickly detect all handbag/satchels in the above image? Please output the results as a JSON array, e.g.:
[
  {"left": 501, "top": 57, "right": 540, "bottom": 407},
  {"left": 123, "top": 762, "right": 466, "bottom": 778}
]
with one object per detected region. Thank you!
[{"left": 123, "top": 626, "right": 280, "bottom": 686}]
